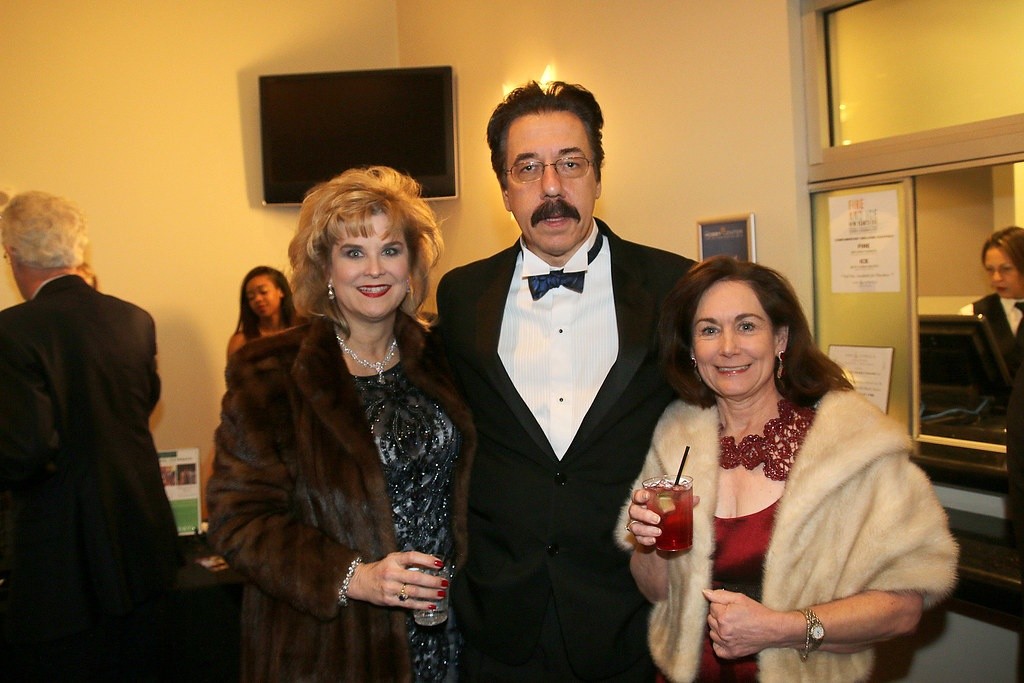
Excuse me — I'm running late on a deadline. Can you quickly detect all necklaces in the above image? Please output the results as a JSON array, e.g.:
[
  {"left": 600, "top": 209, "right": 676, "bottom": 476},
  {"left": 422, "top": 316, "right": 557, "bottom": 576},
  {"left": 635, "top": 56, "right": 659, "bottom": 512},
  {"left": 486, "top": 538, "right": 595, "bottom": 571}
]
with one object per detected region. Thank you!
[{"left": 337, "top": 334, "right": 397, "bottom": 384}]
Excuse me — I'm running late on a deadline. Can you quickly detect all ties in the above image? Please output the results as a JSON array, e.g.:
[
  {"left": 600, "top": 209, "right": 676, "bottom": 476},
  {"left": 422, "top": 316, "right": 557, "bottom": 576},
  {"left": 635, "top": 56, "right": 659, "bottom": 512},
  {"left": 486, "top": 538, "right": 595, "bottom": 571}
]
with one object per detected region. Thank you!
[{"left": 1015, "top": 301, "right": 1023, "bottom": 343}]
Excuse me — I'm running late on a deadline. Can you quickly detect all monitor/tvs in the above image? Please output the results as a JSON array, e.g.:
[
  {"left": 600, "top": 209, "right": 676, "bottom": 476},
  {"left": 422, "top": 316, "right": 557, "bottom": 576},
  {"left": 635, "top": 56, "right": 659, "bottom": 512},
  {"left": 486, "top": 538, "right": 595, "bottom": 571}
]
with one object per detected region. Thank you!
[
  {"left": 919, "top": 312, "right": 1013, "bottom": 413},
  {"left": 259, "top": 65, "right": 457, "bottom": 207}
]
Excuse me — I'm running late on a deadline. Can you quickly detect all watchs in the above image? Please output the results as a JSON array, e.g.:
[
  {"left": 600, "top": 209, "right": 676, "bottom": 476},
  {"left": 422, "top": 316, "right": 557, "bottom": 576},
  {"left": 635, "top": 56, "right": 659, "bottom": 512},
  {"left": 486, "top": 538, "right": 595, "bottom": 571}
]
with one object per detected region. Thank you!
[{"left": 795, "top": 608, "right": 824, "bottom": 653}]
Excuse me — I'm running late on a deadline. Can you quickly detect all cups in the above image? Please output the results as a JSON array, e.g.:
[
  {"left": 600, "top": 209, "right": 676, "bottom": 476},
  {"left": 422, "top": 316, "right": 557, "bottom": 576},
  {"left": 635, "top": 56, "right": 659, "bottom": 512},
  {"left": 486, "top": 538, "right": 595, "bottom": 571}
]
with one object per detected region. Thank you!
[
  {"left": 642, "top": 476, "right": 693, "bottom": 552},
  {"left": 408, "top": 566, "right": 450, "bottom": 626}
]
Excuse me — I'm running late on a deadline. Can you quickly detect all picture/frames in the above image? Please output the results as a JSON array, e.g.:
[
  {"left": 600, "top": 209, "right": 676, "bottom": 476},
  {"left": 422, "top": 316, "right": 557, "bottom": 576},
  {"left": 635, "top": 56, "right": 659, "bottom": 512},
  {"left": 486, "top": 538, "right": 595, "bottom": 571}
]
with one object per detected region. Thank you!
[{"left": 696, "top": 211, "right": 757, "bottom": 263}]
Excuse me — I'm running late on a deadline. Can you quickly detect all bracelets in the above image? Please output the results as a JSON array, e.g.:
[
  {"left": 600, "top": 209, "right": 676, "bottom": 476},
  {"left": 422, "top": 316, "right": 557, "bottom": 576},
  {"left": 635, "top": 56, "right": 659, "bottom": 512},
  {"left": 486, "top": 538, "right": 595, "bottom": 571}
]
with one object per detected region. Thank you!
[
  {"left": 799, "top": 620, "right": 811, "bottom": 662},
  {"left": 339, "top": 558, "right": 361, "bottom": 608}
]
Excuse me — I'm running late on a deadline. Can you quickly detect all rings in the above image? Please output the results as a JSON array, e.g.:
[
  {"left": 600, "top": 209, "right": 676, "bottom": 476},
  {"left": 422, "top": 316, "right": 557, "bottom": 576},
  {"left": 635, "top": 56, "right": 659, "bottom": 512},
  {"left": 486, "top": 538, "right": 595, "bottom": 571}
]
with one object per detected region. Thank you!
[
  {"left": 398, "top": 584, "right": 408, "bottom": 600},
  {"left": 625, "top": 520, "right": 638, "bottom": 531}
]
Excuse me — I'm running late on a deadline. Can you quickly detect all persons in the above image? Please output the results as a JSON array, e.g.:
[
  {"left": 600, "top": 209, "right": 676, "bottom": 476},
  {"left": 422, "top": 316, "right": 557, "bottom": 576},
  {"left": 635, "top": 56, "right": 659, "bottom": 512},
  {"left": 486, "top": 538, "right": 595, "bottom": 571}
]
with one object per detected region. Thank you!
[
  {"left": 0, "top": 191, "right": 190, "bottom": 683},
  {"left": 614, "top": 255, "right": 957, "bottom": 683},
  {"left": 960, "top": 226, "right": 1024, "bottom": 373},
  {"left": 205, "top": 166, "right": 479, "bottom": 683},
  {"left": 437, "top": 82, "right": 697, "bottom": 683},
  {"left": 227, "top": 265, "right": 295, "bottom": 359}
]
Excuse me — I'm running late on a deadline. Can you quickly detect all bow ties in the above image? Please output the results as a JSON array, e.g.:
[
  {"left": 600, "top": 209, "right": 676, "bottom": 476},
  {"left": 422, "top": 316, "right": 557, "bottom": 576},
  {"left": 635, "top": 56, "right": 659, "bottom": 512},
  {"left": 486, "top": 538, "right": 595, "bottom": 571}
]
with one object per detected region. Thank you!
[{"left": 519, "top": 231, "right": 604, "bottom": 301}]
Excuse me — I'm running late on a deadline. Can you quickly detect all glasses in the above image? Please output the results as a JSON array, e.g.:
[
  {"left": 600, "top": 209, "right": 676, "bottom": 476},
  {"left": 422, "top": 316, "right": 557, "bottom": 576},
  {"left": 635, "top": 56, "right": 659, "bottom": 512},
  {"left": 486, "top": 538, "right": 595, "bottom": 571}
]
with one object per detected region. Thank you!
[{"left": 504, "top": 157, "right": 595, "bottom": 184}]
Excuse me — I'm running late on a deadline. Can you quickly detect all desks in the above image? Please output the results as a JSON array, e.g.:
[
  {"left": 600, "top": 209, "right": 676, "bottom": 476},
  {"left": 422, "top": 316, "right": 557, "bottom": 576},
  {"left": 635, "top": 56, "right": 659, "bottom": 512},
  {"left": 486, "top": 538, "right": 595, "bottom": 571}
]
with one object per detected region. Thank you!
[{"left": 170, "top": 537, "right": 244, "bottom": 683}]
